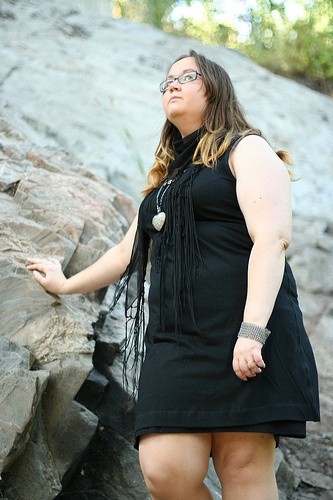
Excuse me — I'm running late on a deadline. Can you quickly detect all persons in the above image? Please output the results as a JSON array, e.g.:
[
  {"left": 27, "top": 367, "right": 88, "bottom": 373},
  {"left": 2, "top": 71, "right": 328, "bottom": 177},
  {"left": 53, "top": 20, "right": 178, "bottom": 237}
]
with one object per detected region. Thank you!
[{"left": 24, "top": 48, "right": 321, "bottom": 500}]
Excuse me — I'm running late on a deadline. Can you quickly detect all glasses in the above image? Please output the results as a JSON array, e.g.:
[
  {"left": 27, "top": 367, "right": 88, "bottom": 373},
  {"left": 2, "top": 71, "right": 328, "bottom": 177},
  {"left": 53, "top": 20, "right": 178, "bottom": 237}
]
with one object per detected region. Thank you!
[{"left": 159, "top": 71, "right": 202, "bottom": 92}]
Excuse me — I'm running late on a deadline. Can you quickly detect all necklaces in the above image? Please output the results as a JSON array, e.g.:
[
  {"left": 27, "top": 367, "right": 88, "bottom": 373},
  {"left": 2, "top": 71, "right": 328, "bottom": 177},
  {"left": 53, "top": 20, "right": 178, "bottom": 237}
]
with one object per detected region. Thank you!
[{"left": 152, "top": 172, "right": 191, "bottom": 231}]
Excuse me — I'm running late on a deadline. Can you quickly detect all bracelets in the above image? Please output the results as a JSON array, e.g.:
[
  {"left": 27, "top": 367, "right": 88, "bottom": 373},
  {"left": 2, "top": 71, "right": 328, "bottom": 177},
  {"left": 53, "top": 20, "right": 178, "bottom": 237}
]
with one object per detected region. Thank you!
[{"left": 236, "top": 322, "right": 271, "bottom": 345}]
